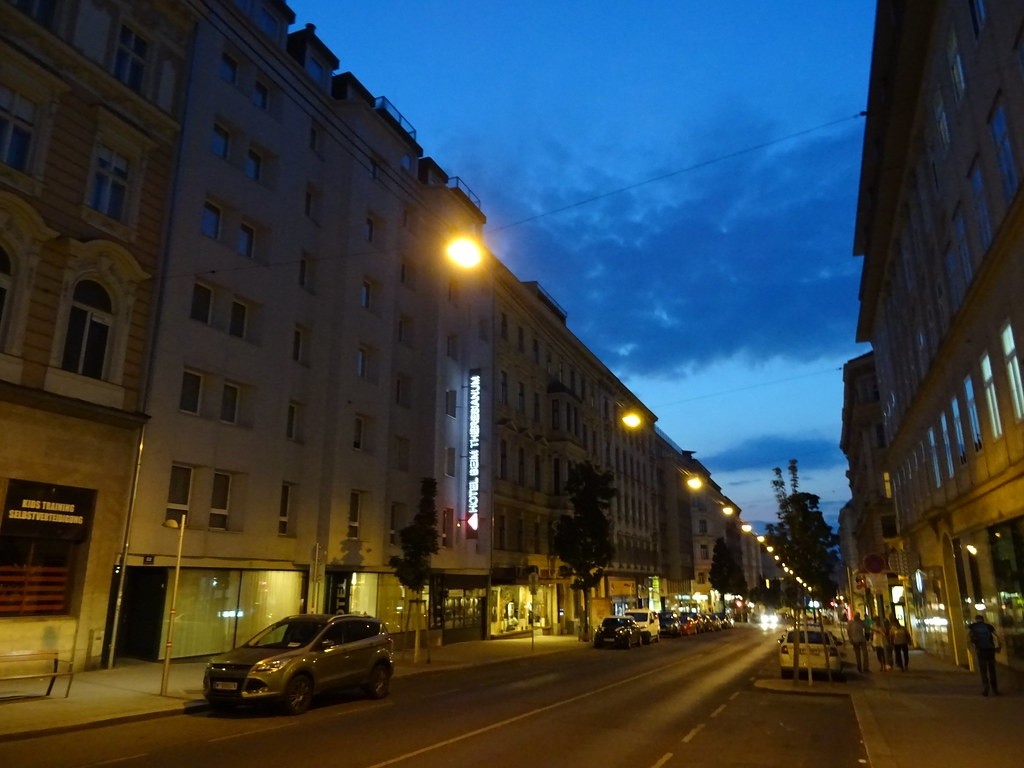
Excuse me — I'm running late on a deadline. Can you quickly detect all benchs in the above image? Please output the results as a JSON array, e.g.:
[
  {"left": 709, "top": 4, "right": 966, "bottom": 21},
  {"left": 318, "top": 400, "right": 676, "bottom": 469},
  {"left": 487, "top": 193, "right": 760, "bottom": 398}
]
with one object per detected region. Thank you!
[{"left": 0, "top": 650, "right": 75, "bottom": 698}]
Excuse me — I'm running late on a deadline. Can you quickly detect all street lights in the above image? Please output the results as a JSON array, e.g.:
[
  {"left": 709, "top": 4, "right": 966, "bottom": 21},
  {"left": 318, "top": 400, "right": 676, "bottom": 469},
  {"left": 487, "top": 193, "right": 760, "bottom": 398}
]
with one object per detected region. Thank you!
[
  {"left": 857, "top": 578, "right": 873, "bottom": 620},
  {"left": 161, "top": 519, "right": 184, "bottom": 696}
]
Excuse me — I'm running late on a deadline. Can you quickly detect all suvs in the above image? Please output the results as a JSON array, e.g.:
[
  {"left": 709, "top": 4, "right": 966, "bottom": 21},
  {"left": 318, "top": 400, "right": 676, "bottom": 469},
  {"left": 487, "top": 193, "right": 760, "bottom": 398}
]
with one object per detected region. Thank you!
[
  {"left": 200, "top": 611, "right": 395, "bottom": 714},
  {"left": 623, "top": 609, "right": 661, "bottom": 644}
]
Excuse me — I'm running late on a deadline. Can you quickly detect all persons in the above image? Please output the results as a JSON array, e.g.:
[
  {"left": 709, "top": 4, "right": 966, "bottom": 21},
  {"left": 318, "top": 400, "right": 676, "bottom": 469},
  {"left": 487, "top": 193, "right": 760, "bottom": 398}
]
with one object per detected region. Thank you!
[
  {"left": 847, "top": 611, "right": 914, "bottom": 674},
  {"left": 507, "top": 598, "right": 517, "bottom": 630},
  {"left": 966, "top": 615, "right": 1005, "bottom": 698}
]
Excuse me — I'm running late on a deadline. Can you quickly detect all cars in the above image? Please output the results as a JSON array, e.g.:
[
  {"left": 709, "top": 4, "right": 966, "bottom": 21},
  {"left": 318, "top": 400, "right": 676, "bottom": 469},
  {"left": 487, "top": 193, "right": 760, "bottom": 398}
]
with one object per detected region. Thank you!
[
  {"left": 593, "top": 615, "right": 644, "bottom": 650},
  {"left": 778, "top": 629, "right": 844, "bottom": 680},
  {"left": 658, "top": 612, "right": 682, "bottom": 636},
  {"left": 787, "top": 614, "right": 820, "bottom": 633},
  {"left": 761, "top": 609, "right": 779, "bottom": 623},
  {"left": 679, "top": 615, "right": 697, "bottom": 636},
  {"left": 683, "top": 613, "right": 733, "bottom": 632}
]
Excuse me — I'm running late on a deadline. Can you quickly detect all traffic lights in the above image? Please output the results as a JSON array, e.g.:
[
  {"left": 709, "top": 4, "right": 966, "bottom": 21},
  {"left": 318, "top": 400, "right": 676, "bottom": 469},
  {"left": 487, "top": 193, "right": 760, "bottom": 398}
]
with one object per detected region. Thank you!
[{"left": 736, "top": 600, "right": 742, "bottom": 608}]
